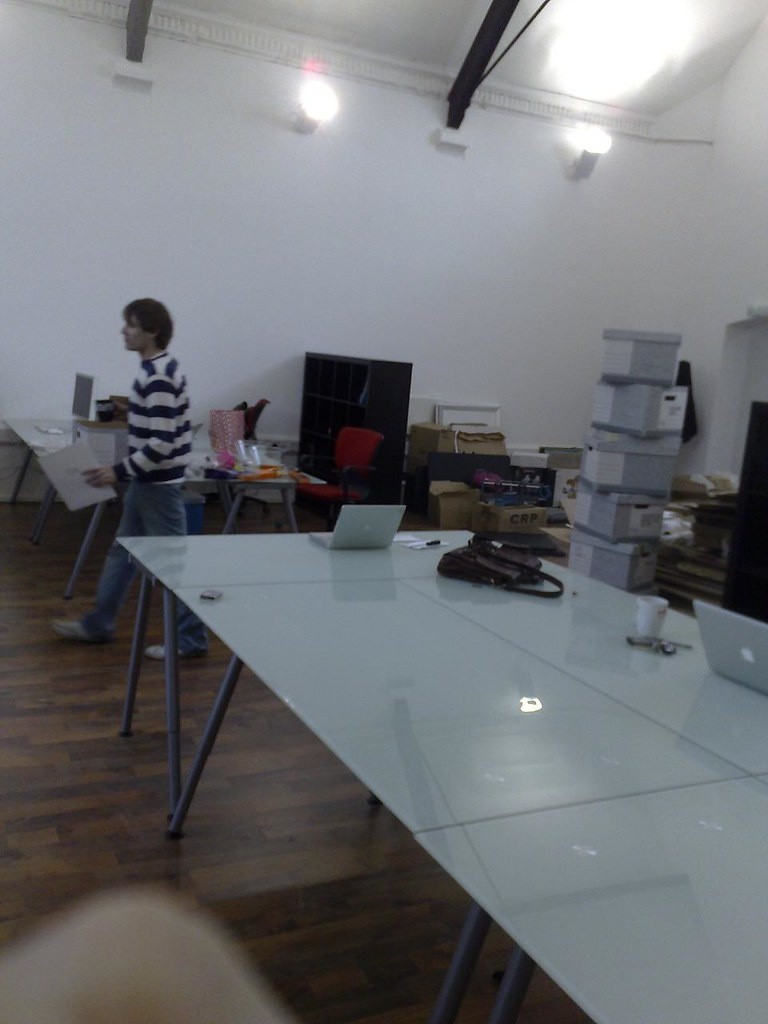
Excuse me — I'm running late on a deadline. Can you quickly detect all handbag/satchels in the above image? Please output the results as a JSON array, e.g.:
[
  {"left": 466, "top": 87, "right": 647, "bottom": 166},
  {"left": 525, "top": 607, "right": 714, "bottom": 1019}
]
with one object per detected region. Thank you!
[{"left": 438, "top": 538, "right": 564, "bottom": 598}]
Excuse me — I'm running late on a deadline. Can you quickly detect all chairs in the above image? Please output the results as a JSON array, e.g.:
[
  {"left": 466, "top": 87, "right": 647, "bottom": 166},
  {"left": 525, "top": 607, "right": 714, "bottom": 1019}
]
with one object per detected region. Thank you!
[{"left": 296, "top": 424, "right": 385, "bottom": 530}]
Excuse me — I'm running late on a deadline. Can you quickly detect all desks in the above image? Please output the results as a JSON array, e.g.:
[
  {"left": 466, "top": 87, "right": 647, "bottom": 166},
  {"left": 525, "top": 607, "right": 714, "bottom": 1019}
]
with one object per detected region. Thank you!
[{"left": 3, "top": 377, "right": 768, "bottom": 1022}]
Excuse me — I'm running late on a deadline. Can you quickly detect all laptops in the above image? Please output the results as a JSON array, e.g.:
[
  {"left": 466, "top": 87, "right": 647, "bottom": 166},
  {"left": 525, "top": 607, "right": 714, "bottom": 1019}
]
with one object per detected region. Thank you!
[
  {"left": 309, "top": 505, "right": 407, "bottom": 549},
  {"left": 693, "top": 599, "right": 768, "bottom": 696}
]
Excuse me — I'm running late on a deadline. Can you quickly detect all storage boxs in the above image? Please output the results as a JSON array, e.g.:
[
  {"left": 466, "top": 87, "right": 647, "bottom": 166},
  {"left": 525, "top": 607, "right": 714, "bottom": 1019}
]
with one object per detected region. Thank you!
[
  {"left": 542, "top": 445, "right": 583, "bottom": 472},
  {"left": 472, "top": 504, "right": 549, "bottom": 532},
  {"left": 428, "top": 480, "right": 482, "bottom": 529},
  {"left": 550, "top": 467, "right": 581, "bottom": 507},
  {"left": 681, "top": 473, "right": 729, "bottom": 500},
  {"left": 567, "top": 528, "right": 662, "bottom": 591},
  {"left": 577, "top": 430, "right": 683, "bottom": 496},
  {"left": 584, "top": 576, "right": 665, "bottom": 600},
  {"left": 589, "top": 385, "right": 689, "bottom": 442},
  {"left": 599, "top": 327, "right": 684, "bottom": 388},
  {"left": 571, "top": 482, "right": 671, "bottom": 543},
  {"left": 407, "top": 424, "right": 506, "bottom": 464}
]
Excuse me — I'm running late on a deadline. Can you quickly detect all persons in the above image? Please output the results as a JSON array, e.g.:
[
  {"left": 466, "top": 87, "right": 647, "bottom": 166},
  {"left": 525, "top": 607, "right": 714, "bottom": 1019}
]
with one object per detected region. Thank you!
[{"left": 52, "top": 298, "right": 210, "bottom": 659}]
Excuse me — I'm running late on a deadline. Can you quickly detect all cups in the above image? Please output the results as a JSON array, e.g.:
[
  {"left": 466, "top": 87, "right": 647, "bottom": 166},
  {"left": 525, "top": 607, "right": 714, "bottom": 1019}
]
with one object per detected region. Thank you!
[
  {"left": 637, "top": 596, "right": 669, "bottom": 641},
  {"left": 238, "top": 439, "right": 285, "bottom": 468}
]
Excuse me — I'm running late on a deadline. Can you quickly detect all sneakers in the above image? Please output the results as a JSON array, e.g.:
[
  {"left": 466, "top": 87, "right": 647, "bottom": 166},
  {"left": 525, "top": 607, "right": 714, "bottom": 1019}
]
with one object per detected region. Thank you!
[
  {"left": 144, "top": 644, "right": 186, "bottom": 661},
  {"left": 52, "top": 620, "right": 91, "bottom": 641}
]
